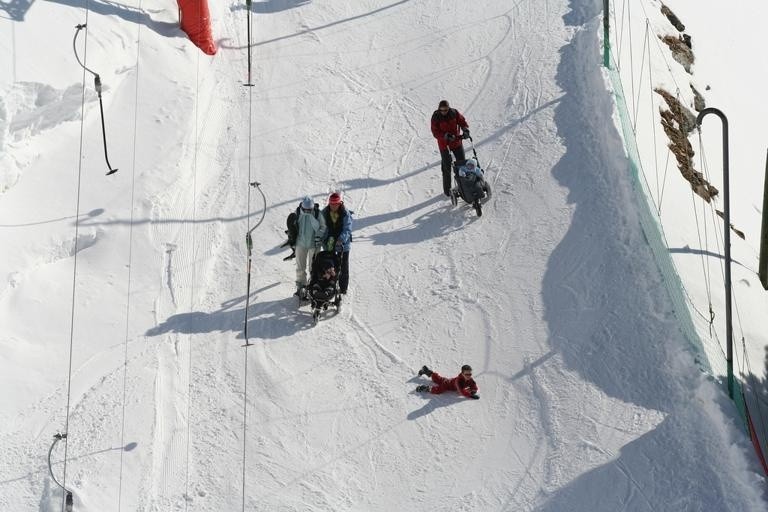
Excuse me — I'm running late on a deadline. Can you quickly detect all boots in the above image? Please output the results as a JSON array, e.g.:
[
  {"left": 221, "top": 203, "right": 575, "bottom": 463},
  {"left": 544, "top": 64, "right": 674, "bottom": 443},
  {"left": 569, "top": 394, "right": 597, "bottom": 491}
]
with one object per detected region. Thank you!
[
  {"left": 418, "top": 365, "right": 432, "bottom": 377},
  {"left": 416, "top": 385, "right": 431, "bottom": 392}
]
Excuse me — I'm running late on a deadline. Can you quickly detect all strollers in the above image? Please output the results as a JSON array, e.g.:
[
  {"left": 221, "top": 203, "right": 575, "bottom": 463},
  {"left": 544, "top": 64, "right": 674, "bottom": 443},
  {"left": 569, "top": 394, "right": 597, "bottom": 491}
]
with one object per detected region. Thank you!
[
  {"left": 446, "top": 133, "right": 494, "bottom": 217},
  {"left": 305, "top": 240, "right": 347, "bottom": 324}
]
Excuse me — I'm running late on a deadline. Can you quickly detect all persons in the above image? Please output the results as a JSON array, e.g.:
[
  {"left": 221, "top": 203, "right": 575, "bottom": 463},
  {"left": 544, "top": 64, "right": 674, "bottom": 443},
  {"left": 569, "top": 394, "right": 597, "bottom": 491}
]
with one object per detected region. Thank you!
[
  {"left": 430, "top": 100, "right": 471, "bottom": 196},
  {"left": 286, "top": 195, "right": 327, "bottom": 301},
  {"left": 458, "top": 158, "right": 488, "bottom": 185},
  {"left": 310, "top": 258, "right": 338, "bottom": 298},
  {"left": 416, "top": 364, "right": 481, "bottom": 399},
  {"left": 319, "top": 192, "right": 354, "bottom": 294}
]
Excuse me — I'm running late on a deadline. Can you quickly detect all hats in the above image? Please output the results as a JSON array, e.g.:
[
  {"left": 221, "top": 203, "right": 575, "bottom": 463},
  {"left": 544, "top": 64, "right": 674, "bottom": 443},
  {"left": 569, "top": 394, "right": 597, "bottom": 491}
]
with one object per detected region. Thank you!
[
  {"left": 303, "top": 195, "right": 314, "bottom": 209},
  {"left": 329, "top": 194, "right": 340, "bottom": 204},
  {"left": 466, "top": 159, "right": 477, "bottom": 167}
]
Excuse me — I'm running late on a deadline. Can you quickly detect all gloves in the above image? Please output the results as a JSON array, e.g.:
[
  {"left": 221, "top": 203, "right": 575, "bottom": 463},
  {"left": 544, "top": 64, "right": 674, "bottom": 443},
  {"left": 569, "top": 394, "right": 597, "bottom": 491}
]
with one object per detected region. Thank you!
[
  {"left": 463, "top": 129, "right": 469, "bottom": 139},
  {"left": 447, "top": 134, "right": 457, "bottom": 142}
]
